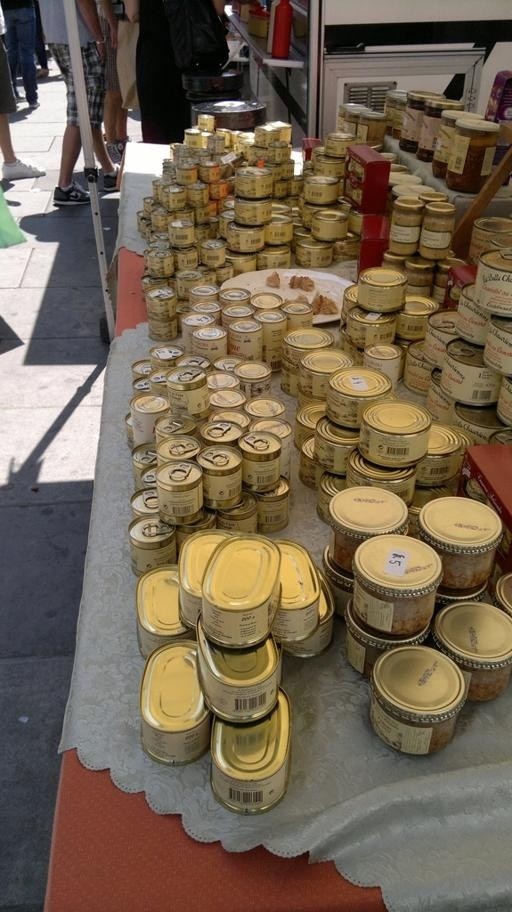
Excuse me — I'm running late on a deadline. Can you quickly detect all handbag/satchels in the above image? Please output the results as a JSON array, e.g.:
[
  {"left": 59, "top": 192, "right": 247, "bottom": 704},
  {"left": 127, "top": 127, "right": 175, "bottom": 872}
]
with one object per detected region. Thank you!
[{"left": 117, "top": 15, "right": 142, "bottom": 110}]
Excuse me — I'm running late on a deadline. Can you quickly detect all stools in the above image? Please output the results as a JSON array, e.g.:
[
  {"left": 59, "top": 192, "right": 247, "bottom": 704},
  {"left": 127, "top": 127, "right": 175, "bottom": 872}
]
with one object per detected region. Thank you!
[
  {"left": 182, "top": 69, "right": 244, "bottom": 101},
  {"left": 192, "top": 102, "right": 266, "bottom": 131}
]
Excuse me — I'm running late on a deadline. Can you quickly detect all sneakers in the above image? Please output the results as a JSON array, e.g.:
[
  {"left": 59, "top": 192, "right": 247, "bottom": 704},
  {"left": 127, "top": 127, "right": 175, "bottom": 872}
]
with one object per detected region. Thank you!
[
  {"left": 118, "top": 138, "right": 129, "bottom": 148},
  {"left": 3, "top": 161, "right": 45, "bottom": 179},
  {"left": 107, "top": 144, "right": 124, "bottom": 164},
  {"left": 103, "top": 166, "right": 123, "bottom": 192},
  {"left": 53, "top": 182, "right": 98, "bottom": 206}
]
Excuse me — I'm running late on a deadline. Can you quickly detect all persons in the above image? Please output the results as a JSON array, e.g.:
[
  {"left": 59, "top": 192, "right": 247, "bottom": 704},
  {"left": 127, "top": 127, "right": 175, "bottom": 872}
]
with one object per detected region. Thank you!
[
  {"left": 0, "top": 0, "right": 41, "bottom": 110},
  {"left": 35, "top": 1, "right": 50, "bottom": 80},
  {"left": 95, "top": 1, "right": 132, "bottom": 165},
  {"left": 124, "top": 0, "right": 224, "bottom": 144},
  {"left": 0, "top": 8, "right": 46, "bottom": 180},
  {"left": 37, "top": 0, "right": 120, "bottom": 205}
]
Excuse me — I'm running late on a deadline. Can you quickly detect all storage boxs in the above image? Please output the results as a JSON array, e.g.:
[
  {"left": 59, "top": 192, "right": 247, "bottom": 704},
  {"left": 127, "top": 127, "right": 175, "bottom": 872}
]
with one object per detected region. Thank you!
[
  {"left": 360, "top": 240, "right": 388, "bottom": 272},
  {"left": 443, "top": 265, "right": 476, "bottom": 307},
  {"left": 339, "top": 206, "right": 391, "bottom": 240},
  {"left": 457, "top": 445, "right": 511, "bottom": 574},
  {"left": 343, "top": 144, "right": 392, "bottom": 213},
  {"left": 302, "top": 139, "right": 321, "bottom": 162}
]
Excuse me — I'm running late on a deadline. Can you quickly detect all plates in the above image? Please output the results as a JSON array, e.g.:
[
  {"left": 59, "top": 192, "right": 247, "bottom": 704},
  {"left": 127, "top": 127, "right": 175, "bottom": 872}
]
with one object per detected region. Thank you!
[{"left": 218, "top": 267, "right": 355, "bottom": 325}]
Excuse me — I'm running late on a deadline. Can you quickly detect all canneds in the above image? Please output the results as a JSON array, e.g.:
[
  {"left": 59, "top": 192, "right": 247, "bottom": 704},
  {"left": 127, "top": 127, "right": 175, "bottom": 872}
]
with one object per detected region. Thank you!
[
  {"left": 414, "top": 423, "right": 462, "bottom": 487},
  {"left": 141, "top": 277, "right": 168, "bottom": 301},
  {"left": 182, "top": 315, "right": 215, "bottom": 354},
  {"left": 220, "top": 131, "right": 258, "bottom": 179},
  {"left": 163, "top": 160, "right": 177, "bottom": 185},
  {"left": 314, "top": 416, "right": 362, "bottom": 477},
  {"left": 127, "top": 515, "right": 177, "bottom": 575},
  {"left": 175, "top": 208, "right": 193, "bottom": 220},
  {"left": 177, "top": 353, "right": 211, "bottom": 374},
  {"left": 322, "top": 545, "right": 356, "bottom": 619},
  {"left": 176, "top": 166, "right": 199, "bottom": 184},
  {"left": 148, "top": 251, "right": 173, "bottom": 277},
  {"left": 193, "top": 326, "right": 226, "bottom": 362},
  {"left": 236, "top": 168, "right": 271, "bottom": 198},
  {"left": 229, "top": 319, "right": 263, "bottom": 363},
  {"left": 207, "top": 136, "right": 224, "bottom": 153},
  {"left": 138, "top": 213, "right": 151, "bottom": 232},
  {"left": 369, "top": 646, "right": 465, "bottom": 754},
  {"left": 273, "top": 202, "right": 291, "bottom": 214},
  {"left": 281, "top": 303, "right": 312, "bottom": 334},
  {"left": 315, "top": 154, "right": 346, "bottom": 178},
  {"left": 475, "top": 249, "right": 511, "bottom": 316},
  {"left": 358, "top": 400, "right": 431, "bottom": 468},
  {"left": 332, "top": 234, "right": 360, "bottom": 265},
  {"left": 497, "top": 377, "right": 512, "bottom": 427},
  {"left": 280, "top": 161, "right": 294, "bottom": 178},
  {"left": 197, "top": 445, "right": 242, "bottom": 509},
  {"left": 211, "top": 685, "right": 292, "bottom": 813},
  {"left": 151, "top": 209, "right": 174, "bottom": 233},
  {"left": 149, "top": 345, "right": 184, "bottom": 371},
  {"left": 456, "top": 284, "right": 492, "bottom": 346},
  {"left": 131, "top": 361, "right": 177, "bottom": 397},
  {"left": 325, "top": 134, "right": 353, "bottom": 156},
  {"left": 140, "top": 641, "right": 211, "bottom": 766},
  {"left": 224, "top": 248, "right": 257, "bottom": 275},
  {"left": 210, "top": 198, "right": 235, "bottom": 238},
  {"left": 244, "top": 398, "right": 288, "bottom": 424},
  {"left": 207, "top": 354, "right": 246, "bottom": 413},
  {"left": 157, "top": 436, "right": 201, "bottom": 465},
  {"left": 218, "top": 491, "right": 257, "bottom": 539},
  {"left": 198, "top": 237, "right": 225, "bottom": 264},
  {"left": 153, "top": 180, "right": 167, "bottom": 201},
  {"left": 329, "top": 487, "right": 409, "bottom": 574},
  {"left": 200, "top": 421, "right": 242, "bottom": 447},
  {"left": 326, "top": 366, "right": 392, "bottom": 429},
  {"left": 178, "top": 508, "right": 217, "bottom": 553},
  {"left": 311, "top": 211, "right": 348, "bottom": 242},
  {"left": 272, "top": 178, "right": 288, "bottom": 199},
  {"left": 280, "top": 561, "right": 334, "bottom": 658},
  {"left": 254, "top": 309, "right": 287, "bottom": 373},
  {"left": 137, "top": 565, "right": 197, "bottom": 658},
  {"left": 146, "top": 287, "right": 175, "bottom": 317},
  {"left": 222, "top": 305, "right": 253, "bottom": 331},
  {"left": 425, "top": 309, "right": 464, "bottom": 367},
  {"left": 456, "top": 429, "right": 473, "bottom": 467},
  {"left": 295, "top": 404, "right": 328, "bottom": 449},
  {"left": 198, "top": 162, "right": 219, "bottom": 183},
  {"left": 268, "top": 142, "right": 288, "bottom": 163},
  {"left": 454, "top": 404, "right": 512, "bottom": 446},
  {"left": 345, "top": 307, "right": 396, "bottom": 348},
  {"left": 265, "top": 120, "right": 291, "bottom": 145},
  {"left": 249, "top": 417, "right": 292, "bottom": 480},
  {"left": 364, "top": 345, "right": 403, "bottom": 385},
  {"left": 281, "top": 329, "right": 333, "bottom": 397},
  {"left": 187, "top": 183, "right": 210, "bottom": 207},
  {"left": 403, "top": 257, "right": 435, "bottom": 299},
  {"left": 154, "top": 414, "right": 198, "bottom": 447},
  {"left": 298, "top": 349, "right": 354, "bottom": 399},
  {"left": 197, "top": 115, "right": 215, "bottom": 132},
  {"left": 227, "top": 221, "right": 265, "bottom": 253},
  {"left": 302, "top": 144, "right": 325, "bottom": 175},
  {"left": 168, "top": 221, "right": 194, "bottom": 245},
  {"left": 289, "top": 177, "right": 303, "bottom": 197},
  {"left": 396, "top": 294, "right": 439, "bottom": 340},
  {"left": 131, "top": 443, "right": 157, "bottom": 490},
  {"left": 195, "top": 609, "right": 280, "bottom": 723},
  {"left": 317, "top": 471, "right": 349, "bottom": 524},
  {"left": 143, "top": 198, "right": 160, "bottom": 215},
  {"left": 406, "top": 484, "right": 451, "bottom": 530},
  {"left": 234, "top": 361, "right": 273, "bottom": 399},
  {"left": 354, "top": 535, "right": 443, "bottom": 634},
  {"left": 193, "top": 204, "right": 210, "bottom": 223},
  {"left": 469, "top": 217, "right": 512, "bottom": 270},
  {"left": 179, "top": 531, "right": 241, "bottom": 630},
  {"left": 210, "top": 182, "right": 228, "bottom": 199},
  {"left": 265, "top": 215, "right": 293, "bottom": 245},
  {"left": 346, "top": 447, "right": 415, "bottom": 506},
  {"left": 170, "top": 247, "right": 198, "bottom": 271},
  {"left": 189, "top": 286, "right": 283, "bottom": 324},
  {"left": 266, "top": 538, "right": 321, "bottom": 642},
  {"left": 238, "top": 432, "right": 281, "bottom": 493},
  {"left": 258, "top": 245, "right": 291, "bottom": 272},
  {"left": 440, "top": 337, "right": 501, "bottom": 407},
  {"left": 433, "top": 602, "right": 512, "bottom": 701},
  {"left": 145, "top": 226, "right": 168, "bottom": 251},
  {"left": 202, "top": 534, "right": 282, "bottom": 647},
  {"left": 496, "top": 573, "right": 512, "bottom": 616},
  {"left": 130, "top": 395, "right": 170, "bottom": 449},
  {"left": 433, "top": 259, "right": 469, "bottom": 302},
  {"left": 295, "top": 240, "right": 332, "bottom": 267},
  {"left": 163, "top": 186, "right": 187, "bottom": 210},
  {"left": 484, "top": 315, "right": 512, "bottom": 377},
  {"left": 234, "top": 196, "right": 273, "bottom": 226},
  {"left": 194, "top": 224, "right": 211, "bottom": 245},
  {"left": 175, "top": 269, "right": 202, "bottom": 298},
  {"left": 198, "top": 262, "right": 234, "bottom": 286},
  {"left": 403, "top": 340, "right": 437, "bottom": 395},
  {"left": 255, "top": 475, "right": 290, "bottom": 533},
  {"left": 345, "top": 597, "right": 430, "bottom": 675},
  {"left": 170, "top": 145, "right": 224, "bottom": 167},
  {"left": 382, "top": 250, "right": 407, "bottom": 272},
  {"left": 426, "top": 368, "right": 459, "bottom": 425},
  {"left": 420, "top": 497, "right": 502, "bottom": 588},
  {"left": 305, "top": 177, "right": 339, "bottom": 204},
  {"left": 148, "top": 317, "right": 178, "bottom": 340},
  {"left": 157, "top": 461, "right": 203, "bottom": 525},
  {"left": 299, "top": 435, "right": 326, "bottom": 489},
  {"left": 183, "top": 127, "right": 204, "bottom": 147},
  {"left": 130, "top": 488, "right": 158, "bottom": 518},
  {"left": 489, "top": 430, "right": 512, "bottom": 446},
  {"left": 303, "top": 203, "right": 335, "bottom": 230},
  {"left": 167, "top": 365, "right": 209, "bottom": 422},
  {"left": 358, "top": 267, "right": 406, "bottom": 313},
  {"left": 264, "top": 164, "right": 282, "bottom": 179},
  {"left": 253, "top": 127, "right": 281, "bottom": 148},
  {"left": 341, "top": 283, "right": 360, "bottom": 322}
]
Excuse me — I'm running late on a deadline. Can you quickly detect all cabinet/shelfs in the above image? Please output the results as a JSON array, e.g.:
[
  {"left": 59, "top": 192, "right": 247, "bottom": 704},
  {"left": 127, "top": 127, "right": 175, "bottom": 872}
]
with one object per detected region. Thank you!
[{"left": 225, "top": 1, "right": 512, "bottom": 146}]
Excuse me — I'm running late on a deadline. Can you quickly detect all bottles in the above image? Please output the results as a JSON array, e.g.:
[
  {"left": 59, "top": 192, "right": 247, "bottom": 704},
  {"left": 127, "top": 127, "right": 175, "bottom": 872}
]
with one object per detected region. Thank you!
[
  {"left": 419, "top": 202, "right": 455, "bottom": 260},
  {"left": 378, "top": 153, "right": 449, "bottom": 206},
  {"left": 446, "top": 120, "right": 500, "bottom": 193},
  {"left": 357, "top": 112, "right": 385, "bottom": 147},
  {"left": 388, "top": 198, "right": 425, "bottom": 257},
  {"left": 337, "top": 103, "right": 361, "bottom": 136},
  {"left": 399, "top": 90, "right": 445, "bottom": 153},
  {"left": 416, "top": 101, "right": 467, "bottom": 161},
  {"left": 393, "top": 98, "right": 410, "bottom": 139},
  {"left": 433, "top": 110, "right": 484, "bottom": 177},
  {"left": 384, "top": 91, "right": 406, "bottom": 134},
  {"left": 344, "top": 107, "right": 371, "bottom": 139}
]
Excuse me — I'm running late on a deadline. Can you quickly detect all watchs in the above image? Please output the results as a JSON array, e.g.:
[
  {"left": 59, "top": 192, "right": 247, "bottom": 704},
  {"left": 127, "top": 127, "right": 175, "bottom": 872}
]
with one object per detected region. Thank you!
[{"left": 95, "top": 36, "right": 107, "bottom": 45}]
